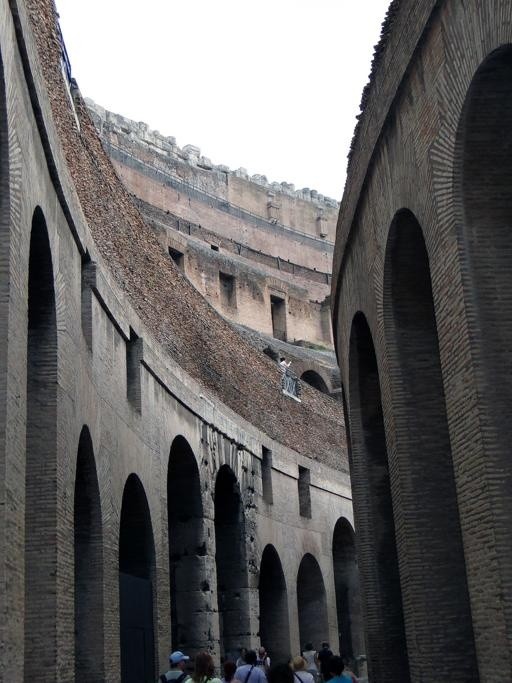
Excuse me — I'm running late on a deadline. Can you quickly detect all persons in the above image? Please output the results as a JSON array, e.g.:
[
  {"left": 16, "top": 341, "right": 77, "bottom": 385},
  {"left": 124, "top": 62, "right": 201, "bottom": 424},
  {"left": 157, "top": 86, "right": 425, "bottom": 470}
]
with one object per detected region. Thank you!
[
  {"left": 158, "top": 641, "right": 369, "bottom": 682},
  {"left": 280, "top": 356, "right": 291, "bottom": 367}
]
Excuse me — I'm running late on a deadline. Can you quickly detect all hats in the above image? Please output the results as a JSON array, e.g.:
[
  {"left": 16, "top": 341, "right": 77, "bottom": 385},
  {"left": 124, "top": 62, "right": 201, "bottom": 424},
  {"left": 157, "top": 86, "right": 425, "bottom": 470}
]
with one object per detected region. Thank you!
[
  {"left": 291, "top": 656, "right": 307, "bottom": 671},
  {"left": 169, "top": 651, "right": 190, "bottom": 664}
]
